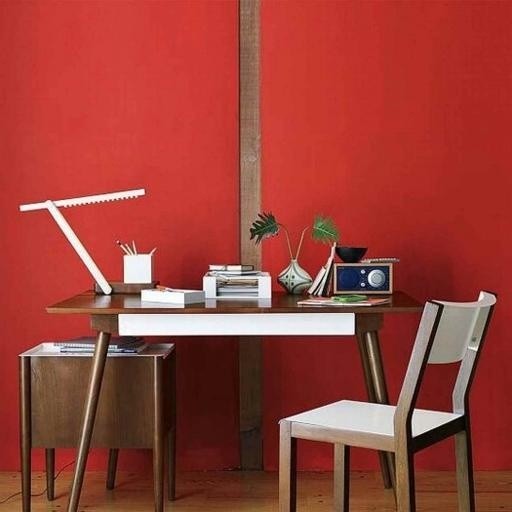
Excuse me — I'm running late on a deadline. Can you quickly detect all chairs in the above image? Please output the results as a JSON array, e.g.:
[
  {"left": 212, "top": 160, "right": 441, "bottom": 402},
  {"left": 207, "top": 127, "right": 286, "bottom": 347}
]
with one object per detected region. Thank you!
[{"left": 279, "top": 291, "right": 497, "bottom": 512}]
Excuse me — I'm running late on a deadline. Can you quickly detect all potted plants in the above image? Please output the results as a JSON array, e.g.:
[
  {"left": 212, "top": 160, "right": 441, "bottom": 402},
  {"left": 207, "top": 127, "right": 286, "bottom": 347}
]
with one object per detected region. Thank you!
[{"left": 251, "top": 211, "right": 340, "bottom": 294}]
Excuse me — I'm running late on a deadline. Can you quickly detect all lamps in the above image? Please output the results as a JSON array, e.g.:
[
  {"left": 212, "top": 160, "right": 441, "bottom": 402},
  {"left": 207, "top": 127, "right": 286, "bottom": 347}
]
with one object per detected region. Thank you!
[{"left": 18, "top": 189, "right": 160, "bottom": 296}]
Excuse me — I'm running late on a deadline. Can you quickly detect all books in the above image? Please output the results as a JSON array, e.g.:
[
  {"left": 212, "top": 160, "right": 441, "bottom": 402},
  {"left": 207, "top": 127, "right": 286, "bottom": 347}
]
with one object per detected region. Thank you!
[
  {"left": 52, "top": 338, "right": 146, "bottom": 354},
  {"left": 297, "top": 296, "right": 390, "bottom": 307},
  {"left": 207, "top": 263, "right": 255, "bottom": 272}
]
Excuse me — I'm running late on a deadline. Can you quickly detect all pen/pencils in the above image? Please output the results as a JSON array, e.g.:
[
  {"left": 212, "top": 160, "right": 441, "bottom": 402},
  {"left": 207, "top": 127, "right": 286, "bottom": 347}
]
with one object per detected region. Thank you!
[
  {"left": 124, "top": 243, "right": 133, "bottom": 255},
  {"left": 156, "top": 284, "right": 175, "bottom": 292},
  {"left": 116, "top": 240, "right": 131, "bottom": 255},
  {"left": 132, "top": 240, "right": 137, "bottom": 254},
  {"left": 149, "top": 247, "right": 157, "bottom": 255}
]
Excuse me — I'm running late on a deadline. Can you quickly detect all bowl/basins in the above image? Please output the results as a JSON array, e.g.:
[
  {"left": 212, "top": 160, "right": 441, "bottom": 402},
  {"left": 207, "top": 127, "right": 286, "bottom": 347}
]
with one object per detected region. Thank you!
[{"left": 335, "top": 247, "right": 368, "bottom": 263}]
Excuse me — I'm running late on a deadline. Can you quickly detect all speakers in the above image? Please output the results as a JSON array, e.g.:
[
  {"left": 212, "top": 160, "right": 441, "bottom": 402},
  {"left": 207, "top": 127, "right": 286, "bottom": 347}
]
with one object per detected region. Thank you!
[{"left": 333, "top": 263, "right": 392, "bottom": 296}]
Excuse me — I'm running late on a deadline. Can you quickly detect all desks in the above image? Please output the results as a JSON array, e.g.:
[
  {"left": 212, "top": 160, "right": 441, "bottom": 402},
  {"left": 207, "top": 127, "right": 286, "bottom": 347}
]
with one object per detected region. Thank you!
[
  {"left": 45, "top": 290, "right": 425, "bottom": 512},
  {"left": 18, "top": 341, "right": 177, "bottom": 511}
]
